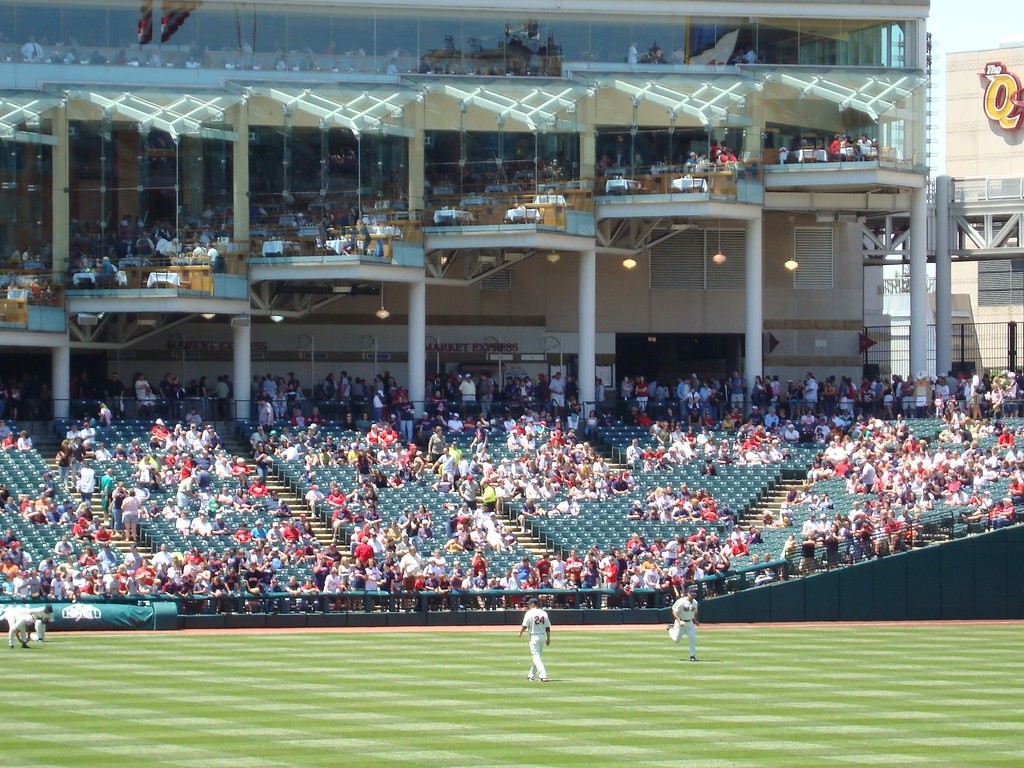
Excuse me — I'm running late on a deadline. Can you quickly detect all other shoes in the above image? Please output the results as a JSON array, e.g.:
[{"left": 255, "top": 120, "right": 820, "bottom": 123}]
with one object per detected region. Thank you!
[
  {"left": 667, "top": 624, "right": 673, "bottom": 630},
  {"left": 690, "top": 656, "right": 696, "bottom": 661}
]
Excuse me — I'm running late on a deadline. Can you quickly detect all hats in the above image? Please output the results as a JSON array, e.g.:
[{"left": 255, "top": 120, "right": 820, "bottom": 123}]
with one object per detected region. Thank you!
[
  {"left": 1002, "top": 497, "right": 1013, "bottom": 507},
  {"left": 272, "top": 521, "right": 279, "bottom": 527},
  {"left": 21, "top": 572, "right": 29, "bottom": 578},
  {"left": 311, "top": 485, "right": 320, "bottom": 490},
  {"left": 42, "top": 569, "right": 52, "bottom": 575},
  {"left": 467, "top": 475, "right": 473, "bottom": 484},
  {"left": 215, "top": 514, "right": 222, "bottom": 519},
  {"left": 44, "top": 473, "right": 53, "bottom": 478},
  {"left": 190, "top": 423, "right": 196, "bottom": 428},
  {"left": 355, "top": 525, "right": 363, "bottom": 533},
  {"left": 31, "top": 570, "right": 37, "bottom": 576},
  {"left": 887, "top": 467, "right": 898, "bottom": 473},
  {"left": 44, "top": 605, "right": 53, "bottom": 613},
  {"left": 528, "top": 599, "right": 540, "bottom": 605},
  {"left": 688, "top": 586, "right": 700, "bottom": 598},
  {"left": 252, "top": 370, "right": 1023, "bottom": 463},
  {"left": 117, "top": 444, "right": 123, "bottom": 452},
  {"left": 167, "top": 498, "right": 173, "bottom": 502},
  {"left": 155, "top": 417, "right": 164, "bottom": 425}
]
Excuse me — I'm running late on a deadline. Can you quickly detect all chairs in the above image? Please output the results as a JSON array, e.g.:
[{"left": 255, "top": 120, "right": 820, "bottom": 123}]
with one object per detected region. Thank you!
[
  {"left": 801, "top": 146, "right": 816, "bottom": 163},
  {"left": 0, "top": 398, "right": 1024, "bottom": 603},
  {"left": 826, "top": 147, "right": 842, "bottom": 162},
  {"left": 780, "top": 147, "right": 794, "bottom": 163},
  {"left": 844, "top": 147, "right": 917, "bottom": 164}
]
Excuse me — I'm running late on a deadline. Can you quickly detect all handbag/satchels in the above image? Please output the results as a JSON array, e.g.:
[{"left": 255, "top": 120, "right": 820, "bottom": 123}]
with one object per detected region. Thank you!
[
  {"left": 99, "top": 416, "right": 107, "bottom": 426},
  {"left": 58, "top": 456, "right": 70, "bottom": 468}
]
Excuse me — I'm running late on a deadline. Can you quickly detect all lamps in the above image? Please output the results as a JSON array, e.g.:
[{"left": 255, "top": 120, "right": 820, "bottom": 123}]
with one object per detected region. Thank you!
[
  {"left": 785, "top": 216, "right": 798, "bottom": 271},
  {"left": 375, "top": 281, "right": 390, "bottom": 320},
  {"left": 547, "top": 250, "right": 561, "bottom": 263},
  {"left": 713, "top": 227, "right": 727, "bottom": 263},
  {"left": 441, "top": 256, "right": 447, "bottom": 264},
  {"left": 623, "top": 258, "right": 637, "bottom": 270},
  {"left": 200, "top": 313, "right": 215, "bottom": 320}
]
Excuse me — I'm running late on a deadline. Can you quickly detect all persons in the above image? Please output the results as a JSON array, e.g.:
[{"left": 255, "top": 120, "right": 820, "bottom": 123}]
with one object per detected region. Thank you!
[
  {"left": 667, "top": 587, "right": 700, "bottom": 661},
  {"left": 0, "top": 40, "right": 1024, "bottom": 650},
  {"left": 518, "top": 598, "right": 551, "bottom": 682}
]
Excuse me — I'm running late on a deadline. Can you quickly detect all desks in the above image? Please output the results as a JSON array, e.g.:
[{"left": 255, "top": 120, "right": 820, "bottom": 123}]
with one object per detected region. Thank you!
[
  {"left": 261, "top": 240, "right": 301, "bottom": 257},
  {"left": 215, "top": 242, "right": 234, "bottom": 252},
  {"left": 26, "top": 262, "right": 45, "bottom": 269},
  {"left": 17, "top": 275, "right": 43, "bottom": 285},
  {"left": 250, "top": 228, "right": 274, "bottom": 237},
  {"left": 279, "top": 214, "right": 294, "bottom": 225},
  {"left": 0, "top": 275, "right": 16, "bottom": 288},
  {"left": 72, "top": 271, "right": 125, "bottom": 290},
  {"left": 361, "top": 214, "right": 387, "bottom": 224},
  {"left": 326, "top": 239, "right": 364, "bottom": 256},
  {"left": 171, "top": 257, "right": 211, "bottom": 264},
  {"left": 120, "top": 258, "right": 148, "bottom": 267},
  {"left": 148, "top": 272, "right": 180, "bottom": 289},
  {"left": 370, "top": 226, "right": 402, "bottom": 240},
  {"left": 162, "top": 243, "right": 192, "bottom": 254},
  {"left": 433, "top": 144, "right": 858, "bottom": 222},
  {"left": 348, "top": 234, "right": 402, "bottom": 258},
  {"left": 605, "top": 180, "right": 639, "bottom": 194},
  {"left": 308, "top": 203, "right": 333, "bottom": 209},
  {"left": 300, "top": 228, "right": 324, "bottom": 236},
  {"left": 8, "top": 290, "right": 44, "bottom": 305}
]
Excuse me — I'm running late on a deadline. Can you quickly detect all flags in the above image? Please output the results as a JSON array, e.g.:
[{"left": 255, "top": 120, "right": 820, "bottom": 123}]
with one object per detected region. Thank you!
[{"left": 137, "top": 0, "right": 202, "bottom": 45}]
